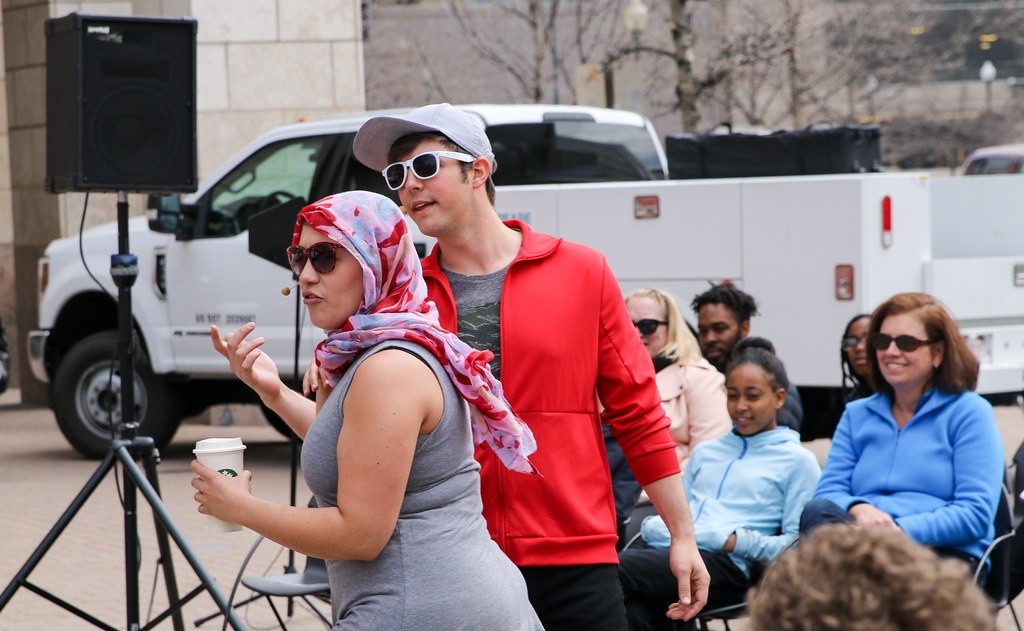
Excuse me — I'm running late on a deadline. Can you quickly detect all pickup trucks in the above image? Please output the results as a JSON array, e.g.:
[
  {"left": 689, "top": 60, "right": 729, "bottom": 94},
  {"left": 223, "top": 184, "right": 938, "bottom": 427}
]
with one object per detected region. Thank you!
[{"left": 24, "top": 104, "right": 1022, "bottom": 460}]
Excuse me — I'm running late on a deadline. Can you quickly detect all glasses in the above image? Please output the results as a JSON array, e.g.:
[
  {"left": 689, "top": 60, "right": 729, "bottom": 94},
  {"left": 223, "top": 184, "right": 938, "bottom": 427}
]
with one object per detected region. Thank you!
[
  {"left": 633, "top": 319, "right": 669, "bottom": 337},
  {"left": 874, "top": 334, "right": 934, "bottom": 352},
  {"left": 286, "top": 242, "right": 344, "bottom": 276},
  {"left": 381, "top": 150, "right": 476, "bottom": 190},
  {"left": 842, "top": 334, "right": 870, "bottom": 349}
]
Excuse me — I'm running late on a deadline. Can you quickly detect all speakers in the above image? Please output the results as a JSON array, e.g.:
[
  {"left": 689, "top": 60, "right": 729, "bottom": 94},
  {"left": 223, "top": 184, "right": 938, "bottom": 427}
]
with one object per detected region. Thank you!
[{"left": 45, "top": 12, "right": 198, "bottom": 195}]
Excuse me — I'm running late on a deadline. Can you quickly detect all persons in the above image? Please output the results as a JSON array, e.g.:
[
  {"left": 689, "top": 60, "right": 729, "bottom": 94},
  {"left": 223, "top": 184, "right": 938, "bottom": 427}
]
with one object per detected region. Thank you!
[
  {"left": 840, "top": 314, "right": 877, "bottom": 401},
  {"left": 691, "top": 287, "right": 802, "bottom": 427},
  {"left": 751, "top": 523, "right": 994, "bottom": 631},
  {"left": 191, "top": 189, "right": 546, "bottom": 631},
  {"left": 210, "top": 103, "right": 710, "bottom": 631},
  {"left": 601, "top": 290, "right": 733, "bottom": 525},
  {"left": 616, "top": 337, "right": 821, "bottom": 631},
  {"left": 799, "top": 292, "right": 1005, "bottom": 581}
]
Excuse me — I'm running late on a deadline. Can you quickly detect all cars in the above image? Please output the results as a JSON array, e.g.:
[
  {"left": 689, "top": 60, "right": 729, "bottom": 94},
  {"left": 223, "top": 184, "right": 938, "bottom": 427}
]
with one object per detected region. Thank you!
[{"left": 959, "top": 142, "right": 1023, "bottom": 173}]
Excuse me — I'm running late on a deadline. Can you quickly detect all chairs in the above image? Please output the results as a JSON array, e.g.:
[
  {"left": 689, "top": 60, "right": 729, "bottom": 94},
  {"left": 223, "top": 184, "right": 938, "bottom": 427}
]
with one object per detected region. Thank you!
[
  {"left": 242, "top": 498, "right": 332, "bottom": 631},
  {"left": 697, "top": 484, "right": 1022, "bottom": 631}
]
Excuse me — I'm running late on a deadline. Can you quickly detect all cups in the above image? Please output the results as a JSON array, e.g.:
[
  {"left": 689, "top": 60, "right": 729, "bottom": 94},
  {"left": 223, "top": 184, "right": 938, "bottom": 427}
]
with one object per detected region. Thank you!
[{"left": 191, "top": 436, "right": 247, "bottom": 531}]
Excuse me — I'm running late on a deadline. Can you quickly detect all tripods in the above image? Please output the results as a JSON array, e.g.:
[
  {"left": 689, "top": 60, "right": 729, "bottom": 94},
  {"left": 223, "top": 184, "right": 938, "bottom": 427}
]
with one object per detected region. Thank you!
[{"left": 0, "top": 193, "right": 334, "bottom": 631}]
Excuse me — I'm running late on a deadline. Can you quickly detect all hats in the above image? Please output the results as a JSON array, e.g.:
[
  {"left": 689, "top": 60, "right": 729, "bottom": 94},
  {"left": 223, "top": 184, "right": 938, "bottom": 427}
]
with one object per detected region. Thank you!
[{"left": 352, "top": 103, "right": 495, "bottom": 173}]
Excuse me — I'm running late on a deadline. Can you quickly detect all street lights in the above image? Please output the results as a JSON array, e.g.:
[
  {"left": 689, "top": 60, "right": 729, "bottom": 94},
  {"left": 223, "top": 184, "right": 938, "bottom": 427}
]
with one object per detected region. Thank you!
[
  {"left": 623, "top": 0, "right": 651, "bottom": 114},
  {"left": 980, "top": 60, "right": 998, "bottom": 110}
]
